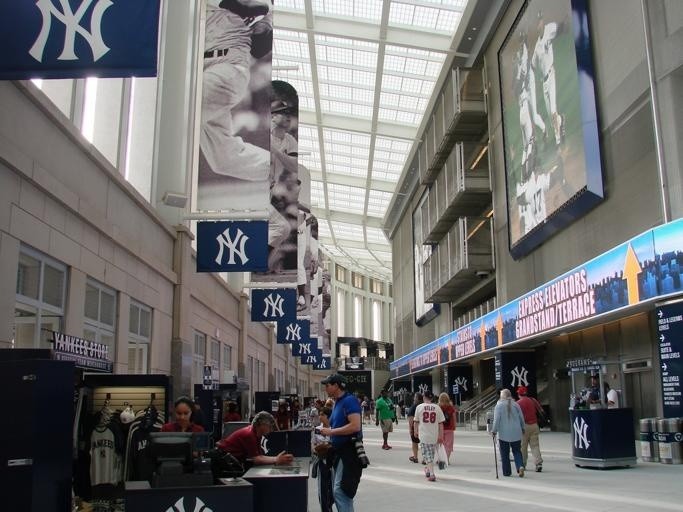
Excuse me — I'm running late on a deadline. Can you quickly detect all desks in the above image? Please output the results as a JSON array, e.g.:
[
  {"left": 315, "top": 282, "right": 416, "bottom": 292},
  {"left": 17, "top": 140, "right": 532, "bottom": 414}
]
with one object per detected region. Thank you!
[
  {"left": 122, "top": 477, "right": 254, "bottom": 512},
  {"left": 243, "top": 455, "right": 309, "bottom": 512}
]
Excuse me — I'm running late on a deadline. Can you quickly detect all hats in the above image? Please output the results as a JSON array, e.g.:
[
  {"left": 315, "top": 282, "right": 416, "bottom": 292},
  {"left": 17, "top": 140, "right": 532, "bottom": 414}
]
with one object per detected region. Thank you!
[
  {"left": 517, "top": 386, "right": 527, "bottom": 394},
  {"left": 320, "top": 375, "right": 342, "bottom": 384},
  {"left": 591, "top": 373, "right": 599, "bottom": 378}
]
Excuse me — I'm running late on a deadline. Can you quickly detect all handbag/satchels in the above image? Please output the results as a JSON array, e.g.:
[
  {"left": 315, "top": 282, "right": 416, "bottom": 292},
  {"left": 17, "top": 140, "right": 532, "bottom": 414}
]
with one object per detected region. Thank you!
[{"left": 536, "top": 410, "right": 553, "bottom": 427}]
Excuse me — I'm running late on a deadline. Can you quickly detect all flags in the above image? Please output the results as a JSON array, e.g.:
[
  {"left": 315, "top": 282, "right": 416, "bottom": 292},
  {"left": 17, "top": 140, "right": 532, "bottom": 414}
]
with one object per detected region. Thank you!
[{"left": 196, "top": 1, "right": 331, "bottom": 370}]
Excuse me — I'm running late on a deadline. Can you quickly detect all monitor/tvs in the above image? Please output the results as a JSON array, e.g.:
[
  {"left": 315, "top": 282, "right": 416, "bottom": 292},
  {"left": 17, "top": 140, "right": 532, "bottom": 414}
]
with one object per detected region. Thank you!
[{"left": 150, "top": 433, "right": 194, "bottom": 474}]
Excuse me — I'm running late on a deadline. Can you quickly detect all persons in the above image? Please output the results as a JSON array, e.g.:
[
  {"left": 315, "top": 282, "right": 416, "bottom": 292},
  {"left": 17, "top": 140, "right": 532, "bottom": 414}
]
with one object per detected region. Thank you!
[
  {"left": 218, "top": 373, "right": 369, "bottom": 510},
  {"left": 509, "top": 11, "right": 571, "bottom": 237},
  {"left": 161, "top": 396, "right": 204, "bottom": 512},
  {"left": 576, "top": 375, "right": 617, "bottom": 409},
  {"left": 491, "top": 387, "right": 548, "bottom": 477},
  {"left": 364, "top": 389, "right": 456, "bottom": 480}
]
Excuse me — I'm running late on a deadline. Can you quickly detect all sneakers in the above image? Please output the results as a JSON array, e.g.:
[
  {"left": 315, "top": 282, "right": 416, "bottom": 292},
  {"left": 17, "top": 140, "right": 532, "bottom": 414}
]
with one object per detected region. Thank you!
[
  {"left": 409, "top": 456, "right": 436, "bottom": 481},
  {"left": 382, "top": 444, "right": 392, "bottom": 450},
  {"left": 535, "top": 464, "right": 543, "bottom": 472},
  {"left": 519, "top": 467, "right": 524, "bottom": 477}
]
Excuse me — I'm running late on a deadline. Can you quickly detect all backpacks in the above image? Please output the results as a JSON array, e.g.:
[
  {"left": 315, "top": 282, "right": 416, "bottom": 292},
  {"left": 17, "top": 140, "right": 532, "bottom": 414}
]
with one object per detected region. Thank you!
[{"left": 441, "top": 406, "right": 450, "bottom": 424}]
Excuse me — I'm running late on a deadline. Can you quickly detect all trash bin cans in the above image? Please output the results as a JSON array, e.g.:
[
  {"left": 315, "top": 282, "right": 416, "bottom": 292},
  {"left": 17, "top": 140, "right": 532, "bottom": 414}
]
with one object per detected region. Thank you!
[
  {"left": 405, "top": 408, "right": 410, "bottom": 419},
  {"left": 486, "top": 411, "right": 495, "bottom": 433},
  {"left": 639, "top": 417, "right": 662, "bottom": 462},
  {"left": 656, "top": 417, "right": 683, "bottom": 465}
]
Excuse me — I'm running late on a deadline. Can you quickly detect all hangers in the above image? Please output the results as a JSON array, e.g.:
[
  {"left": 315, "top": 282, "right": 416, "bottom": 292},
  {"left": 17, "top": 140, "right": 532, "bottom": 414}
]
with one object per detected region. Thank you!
[{"left": 96, "top": 395, "right": 159, "bottom": 428}]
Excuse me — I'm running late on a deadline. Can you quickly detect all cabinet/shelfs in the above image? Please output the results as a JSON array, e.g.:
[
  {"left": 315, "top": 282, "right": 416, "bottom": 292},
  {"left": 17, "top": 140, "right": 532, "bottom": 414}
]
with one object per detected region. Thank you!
[{"left": 570, "top": 361, "right": 638, "bottom": 469}]
